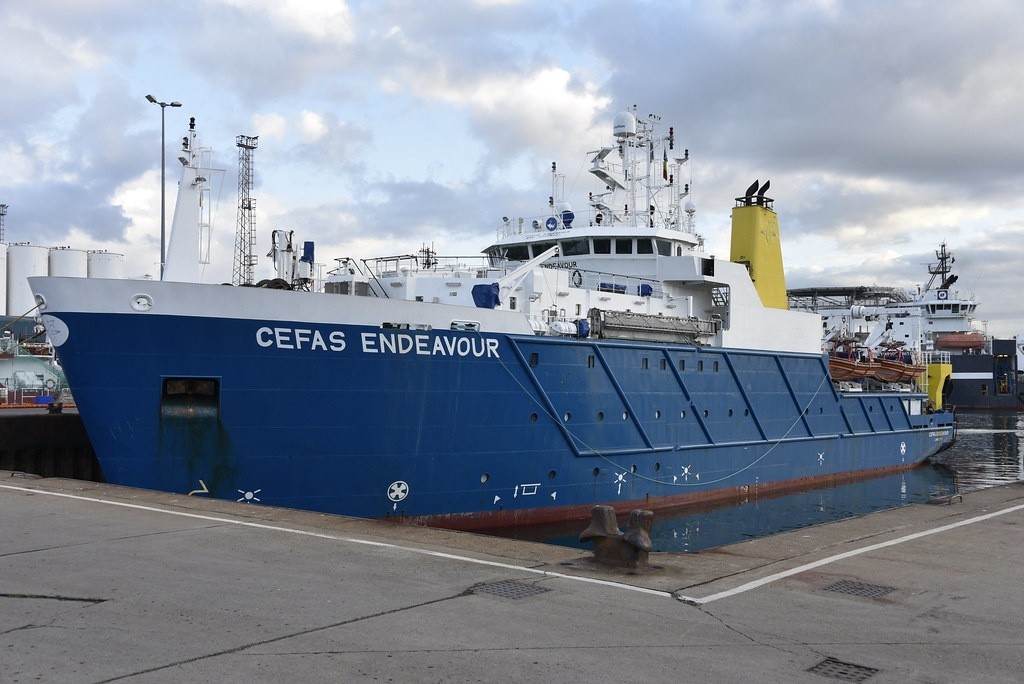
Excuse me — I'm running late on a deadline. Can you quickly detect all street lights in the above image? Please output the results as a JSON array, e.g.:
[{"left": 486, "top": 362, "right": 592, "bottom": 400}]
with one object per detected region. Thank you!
[{"left": 144, "top": 94, "right": 183, "bottom": 279}]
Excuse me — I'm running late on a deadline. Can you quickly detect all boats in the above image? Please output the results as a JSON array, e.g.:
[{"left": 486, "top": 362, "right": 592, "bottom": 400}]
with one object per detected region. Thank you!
[
  {"left": 0, "top": 115, "right": 229, "bottom": 454},
  {"left": 904, "top": 242, "right": 1024, "bottom": 414},
  {"left": 26, "top": 106, "right": 957, "bottom": 555}
]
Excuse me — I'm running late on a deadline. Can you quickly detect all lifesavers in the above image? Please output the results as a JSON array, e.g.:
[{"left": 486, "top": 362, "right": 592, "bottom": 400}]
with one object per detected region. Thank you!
[
  {"left": 939, "top": 292, "right": 946, "bottom": 299},
  {"left": 45, "top": 379, "right": 55, "bottom": 389},
  {"left": 572, "top": 270, "right": 582, "bottom": 287}
]
